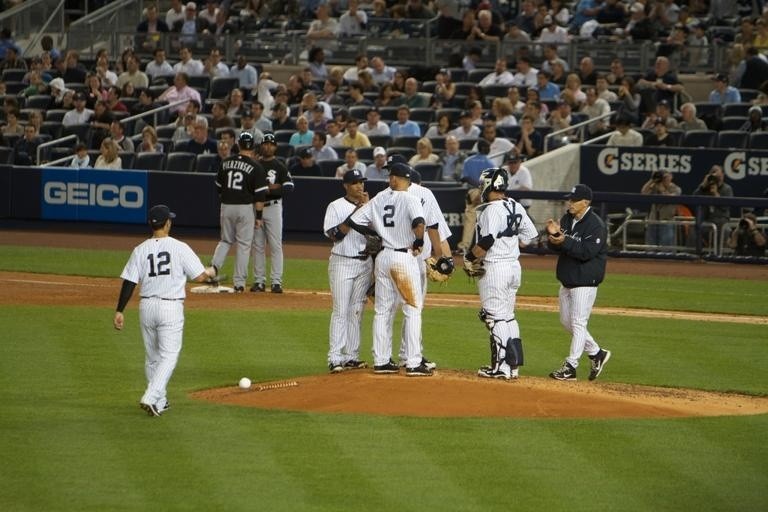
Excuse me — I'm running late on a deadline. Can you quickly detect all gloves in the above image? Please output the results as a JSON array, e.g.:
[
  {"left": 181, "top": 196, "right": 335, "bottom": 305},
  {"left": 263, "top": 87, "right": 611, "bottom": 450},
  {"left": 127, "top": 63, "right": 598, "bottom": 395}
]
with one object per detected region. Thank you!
[{"left": 411, "top": 238, "right": 425, "bottom": 256}]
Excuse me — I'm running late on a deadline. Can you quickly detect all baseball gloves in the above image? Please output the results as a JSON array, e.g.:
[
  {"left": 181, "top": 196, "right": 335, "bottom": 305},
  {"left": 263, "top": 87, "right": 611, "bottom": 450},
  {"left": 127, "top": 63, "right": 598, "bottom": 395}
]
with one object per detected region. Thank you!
[
  {"left": 425, "top": 257, "right": 451, "bottom": 283},
  {"left": 463, "top": 256, "right": 485, "bottom": 276},
  {"left": 358, "top": 234, "right": 381, "bottom": 255}
]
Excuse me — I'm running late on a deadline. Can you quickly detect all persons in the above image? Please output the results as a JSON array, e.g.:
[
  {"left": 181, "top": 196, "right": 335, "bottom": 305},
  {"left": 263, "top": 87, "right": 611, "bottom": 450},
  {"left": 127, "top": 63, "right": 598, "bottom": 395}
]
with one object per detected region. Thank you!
[
  {"left": 203, "top": 132, "right": 264, "bottom": 290},
  {"left": 321, "top": 150, "right": 540, "bottom": 381},
  {"left": 728, "top": 211, "right": 768, "bottom": 256},
  {"left": 641, "top": 170, "right": 683, "bottom": 255},
  {"left": 250, "top": 134, "right": 295, "bottom": 293},
  {"left": 691, "top": 165, "right": 733, "bottom": 245},
  {"left": 2, "top": 1, "right": 768, "bottom": 187},
  {"left": 544, "top": 185, "right": 611, "bottom": 383},
  {"left": 112, "top": 203, "right": 209, "bottom": 417},
  {"left": 454, "top": 138, "right": 493, "bottom": 255}
]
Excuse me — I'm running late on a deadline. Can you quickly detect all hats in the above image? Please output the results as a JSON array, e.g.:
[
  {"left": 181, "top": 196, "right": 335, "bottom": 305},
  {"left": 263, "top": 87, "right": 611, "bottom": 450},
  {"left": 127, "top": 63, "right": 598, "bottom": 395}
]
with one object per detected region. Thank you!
[
  {"left": 342, "top": 168, "right": 368, "bottom": 184},
  {"left": 558, "top": 98, "right": 572, "bottom": 107},
  {"left": 710, "top": 72, "right": 730, "bottom": 86},
  {"left": 656, "top": 99, "right": 672, "bottom": 111},
  {"left": 458, "top": 109, "right": 498, "bottom": 123},
  {"left": 373, "top": 146, "right": 422, "bottom": 184},
  {"left": 541, "top": 14, "right": 556, "bottom": 28},
  {"left": 671, "top": 3, "right": 708, "bottom": 34},
  {"left": 311, "top": 104, "right": 348, "bottom": 118},
  {"left": 562, "top": 184, "right": 594, "bottom": 203},
  {"left": 629, "top": 2, "right": 646, "bottom": 15},
  {"left": 147, "top": 204, "right": 177, "bottom": 226},
  {"left": 70, "top": 91, "right": 88, "bottom": 102},
  {"left": 185, "top": 1, "right": 198, "bottom": 11},
  {"left": 269, "top": 102, "right": 289, "bottom": 113}
]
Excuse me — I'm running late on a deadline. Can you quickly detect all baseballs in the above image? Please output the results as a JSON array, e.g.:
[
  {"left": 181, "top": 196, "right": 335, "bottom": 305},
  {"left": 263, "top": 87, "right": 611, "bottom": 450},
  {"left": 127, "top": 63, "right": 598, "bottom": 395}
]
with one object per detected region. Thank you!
[{"left": 240, "top": 377, "right": 252, "bottom": 389}]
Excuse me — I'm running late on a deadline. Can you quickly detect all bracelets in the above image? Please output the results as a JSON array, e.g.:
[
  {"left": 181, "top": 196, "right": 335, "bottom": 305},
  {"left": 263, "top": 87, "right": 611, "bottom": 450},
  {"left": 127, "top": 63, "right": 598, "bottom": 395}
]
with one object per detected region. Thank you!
[
  {"left": 751, "top": 228, "right": 760, "bottom": 237},
  {"left": 254, "top": 209, "right": 262, "bottom": 221}
]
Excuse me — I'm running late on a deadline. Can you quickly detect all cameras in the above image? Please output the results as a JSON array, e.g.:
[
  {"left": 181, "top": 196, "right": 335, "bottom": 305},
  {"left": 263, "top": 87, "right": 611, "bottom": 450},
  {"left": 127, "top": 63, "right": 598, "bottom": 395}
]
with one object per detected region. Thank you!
[
  {"left": 708, "top": 174, "right": 720, "bottom": 185},
  {"left": 652, "top": 170, "right": 665, "bottom": 184},
  {"left": 738, "top": 218, "right": 750, "bottom": 231}
]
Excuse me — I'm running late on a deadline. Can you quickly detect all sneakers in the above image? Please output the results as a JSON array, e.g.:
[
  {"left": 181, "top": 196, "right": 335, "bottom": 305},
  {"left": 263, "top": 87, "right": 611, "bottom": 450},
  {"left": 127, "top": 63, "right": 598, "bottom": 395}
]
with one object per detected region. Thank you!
[
  {"left": 548, "top": 360, "right": 579, "bottom": 382},
  {"left": 270, "top": 283, "right": 284, "bottom": 293},
  {"left": 586, "top": 348, "right": 611, "bottom": 381},
  {"left": 206, "top": 278, "right": 221, "bottom": 288},
  {"left": 139, "top": 401, "right": 172, "bottom": 418},
  {"left": 249, "top": 282, "right": 266, "bottom": 293},
  {"left": 477, "top": 364, "right": 520, "bottom": 380},
  {"left": 403, "top": 356, "right": 438, "bottom": 377},
  {"left": 328, "top": 359, "right": 368, "bottom": 374},
  {"left": 372, "top": 357, "right": 402, "bottom": 373},
  {"left": 233, "top": 285, "right": 245, "bottom": 293}
]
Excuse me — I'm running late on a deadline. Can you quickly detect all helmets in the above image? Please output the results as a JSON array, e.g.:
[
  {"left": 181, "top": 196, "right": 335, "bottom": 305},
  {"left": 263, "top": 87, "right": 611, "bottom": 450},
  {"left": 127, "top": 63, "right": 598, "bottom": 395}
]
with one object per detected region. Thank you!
[
  {"left": 477, "top": 166, "right": 510, "bottom": 204},
  {"left": 260, "top": 133, "right": 278, "bottom": 145},
  {"left": 238, "top": 132, "right": 254, "bottom": 143}
]
